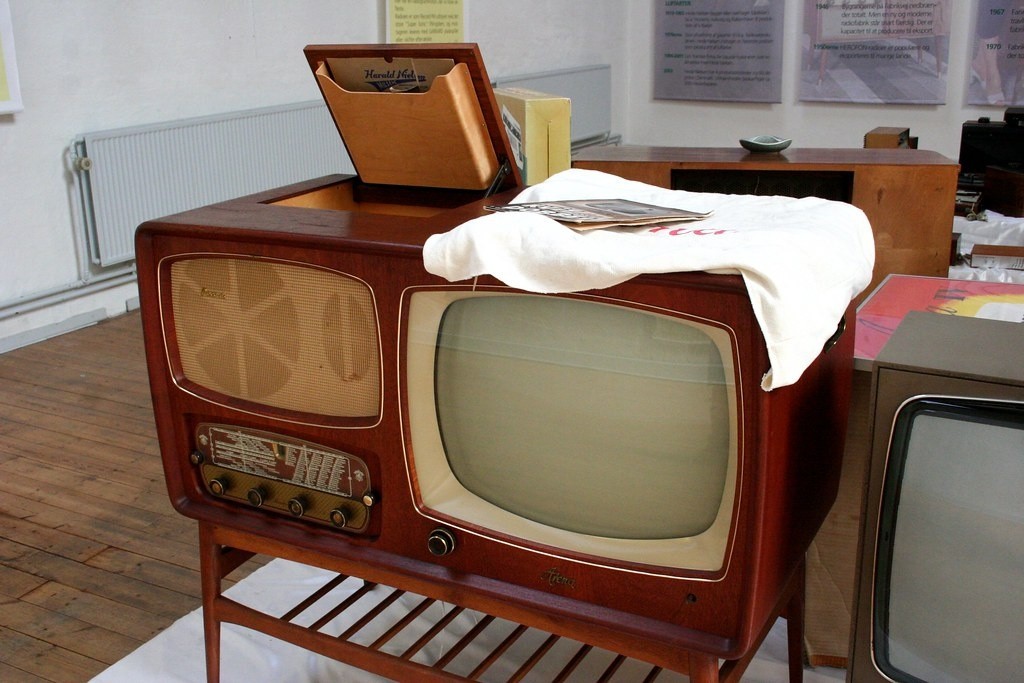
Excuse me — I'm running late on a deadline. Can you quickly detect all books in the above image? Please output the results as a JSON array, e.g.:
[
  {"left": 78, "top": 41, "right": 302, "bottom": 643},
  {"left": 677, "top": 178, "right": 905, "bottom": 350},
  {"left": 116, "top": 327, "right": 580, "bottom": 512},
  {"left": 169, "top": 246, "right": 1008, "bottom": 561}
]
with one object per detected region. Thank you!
[{"left": 482, "top": 197, "right": 715, "bottom": 231}]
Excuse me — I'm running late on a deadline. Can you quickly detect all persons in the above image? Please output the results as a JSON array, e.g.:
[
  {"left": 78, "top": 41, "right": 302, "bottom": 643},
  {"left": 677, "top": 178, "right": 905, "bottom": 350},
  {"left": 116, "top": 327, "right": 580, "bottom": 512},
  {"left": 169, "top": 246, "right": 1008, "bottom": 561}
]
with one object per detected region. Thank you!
[{"left": 969, "top": 1, "right": 1006, "bottom": 106}]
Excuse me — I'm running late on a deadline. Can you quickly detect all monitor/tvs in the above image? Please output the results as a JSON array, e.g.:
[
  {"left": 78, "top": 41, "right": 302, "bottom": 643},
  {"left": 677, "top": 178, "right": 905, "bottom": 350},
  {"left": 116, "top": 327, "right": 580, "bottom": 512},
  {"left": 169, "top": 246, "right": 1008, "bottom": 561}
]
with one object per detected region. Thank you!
[
  {"left": 135, "top": 172, "right": 852, "bottom": 661},
  {"left": 844, "top": 312, "right": 1024, "bottom": 682}
]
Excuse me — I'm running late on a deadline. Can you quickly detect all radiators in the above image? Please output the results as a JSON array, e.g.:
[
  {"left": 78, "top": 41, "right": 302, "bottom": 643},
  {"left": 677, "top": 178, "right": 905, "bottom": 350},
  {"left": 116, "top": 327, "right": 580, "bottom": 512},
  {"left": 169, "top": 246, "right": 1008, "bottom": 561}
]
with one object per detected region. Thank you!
[{"left": 72, "top": 64, "right": 612, "bottom": 267}]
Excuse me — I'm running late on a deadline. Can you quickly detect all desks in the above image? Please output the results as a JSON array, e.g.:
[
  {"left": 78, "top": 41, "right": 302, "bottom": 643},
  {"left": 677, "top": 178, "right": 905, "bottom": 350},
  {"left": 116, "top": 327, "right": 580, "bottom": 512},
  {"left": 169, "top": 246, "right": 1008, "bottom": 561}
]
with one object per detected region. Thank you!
[{"left": 571, "top": 148, "right": 962, "bottom": 279}]
